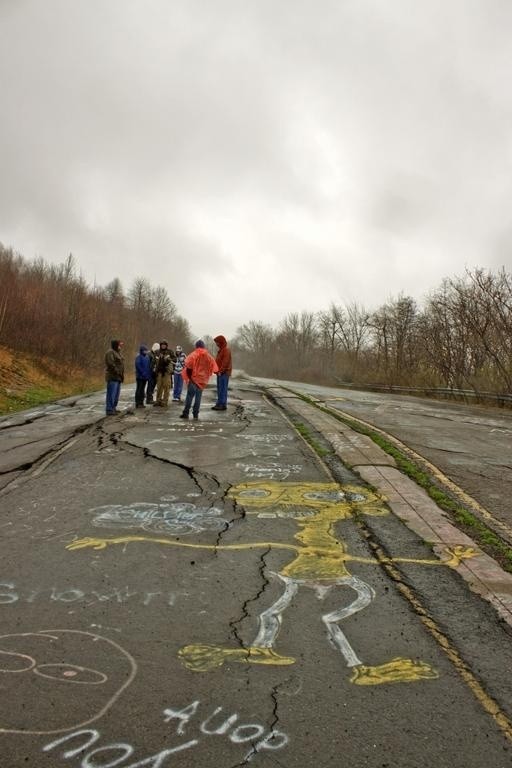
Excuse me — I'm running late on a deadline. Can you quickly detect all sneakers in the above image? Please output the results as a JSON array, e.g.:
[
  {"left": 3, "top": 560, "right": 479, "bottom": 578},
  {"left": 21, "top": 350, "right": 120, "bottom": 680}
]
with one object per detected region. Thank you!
[
  {"left": 146, "top": 399, "right": 168, "bottom": 407},
  {"left": 172, "top": 397, "right": 181, "bottom": 402},
  {"left": 106, "top": 407, "right": 121, "bottom": 416},
  {"left": 211, "top": 405, "right": 227, "bottom": 411},
  {"left": 135, "top": 403, "right": 147, "bottom": 408},
  {"left": 193, "top": 414, "right": 198, "bottom": 419},
  {"left": 179, "top": 413, "right": 189, "bottom": 418}
]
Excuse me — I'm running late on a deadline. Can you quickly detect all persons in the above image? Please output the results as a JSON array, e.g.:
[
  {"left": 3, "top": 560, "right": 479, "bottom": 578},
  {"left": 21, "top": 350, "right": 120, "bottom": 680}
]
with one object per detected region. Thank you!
[
  {"left": 211, "top": 335, "right": 232, "bottom": 410},
  {"left": 179, "top": 339, "right": 219, "bottom": 419},
  {"left": 135, "top": 345, "right": 151, "bottom": 408},
  {"left": 172, "top": 346, "right": 187, "bottom": 402},
  {"left": 153, "top": 339, "right": 177, "bottom": 407},
  {"left": 146, "top": 343, "right": 160, "bottom": 404},
  {"left": 104, "top": 339, "right": 125, "bottom": 415}
]
